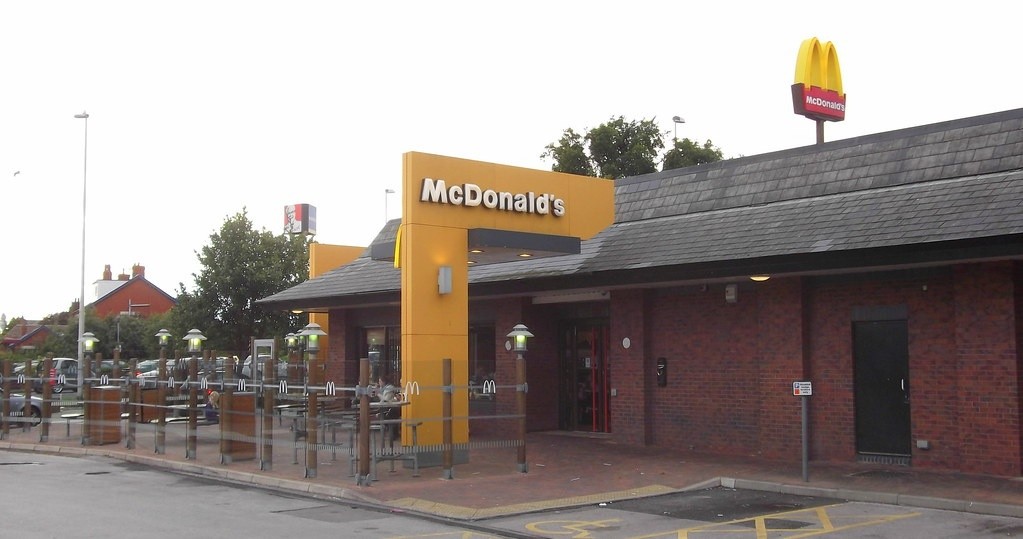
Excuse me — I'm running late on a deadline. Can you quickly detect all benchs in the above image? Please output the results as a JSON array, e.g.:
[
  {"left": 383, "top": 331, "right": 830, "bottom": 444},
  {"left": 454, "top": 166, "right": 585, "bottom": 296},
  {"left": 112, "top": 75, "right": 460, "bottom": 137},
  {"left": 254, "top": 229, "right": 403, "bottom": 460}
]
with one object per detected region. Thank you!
[{"left": 63, "top": 405, "right": 342, "bottom": 452}]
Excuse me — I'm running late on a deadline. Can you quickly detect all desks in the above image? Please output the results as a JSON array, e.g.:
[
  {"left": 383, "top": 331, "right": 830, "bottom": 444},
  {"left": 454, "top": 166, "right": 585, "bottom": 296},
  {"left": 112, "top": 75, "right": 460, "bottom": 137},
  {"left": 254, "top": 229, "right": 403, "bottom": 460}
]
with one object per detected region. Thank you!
[
  {"left": 286, "top": 396, "right": 343, "bottom": 445},
  {"left": 348, "top": 402, "right": 409, "bottom": 462}
]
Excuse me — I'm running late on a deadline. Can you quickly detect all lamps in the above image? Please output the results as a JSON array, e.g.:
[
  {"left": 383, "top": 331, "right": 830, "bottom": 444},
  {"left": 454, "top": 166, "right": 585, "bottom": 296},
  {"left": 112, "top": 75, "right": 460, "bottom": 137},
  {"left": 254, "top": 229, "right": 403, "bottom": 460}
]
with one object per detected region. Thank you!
[
  {"left": 506, "top": 324, "right": 535, "bottom": 354},
  {"left": 81, "top": 320, "right": 329, "bottom": 360}
]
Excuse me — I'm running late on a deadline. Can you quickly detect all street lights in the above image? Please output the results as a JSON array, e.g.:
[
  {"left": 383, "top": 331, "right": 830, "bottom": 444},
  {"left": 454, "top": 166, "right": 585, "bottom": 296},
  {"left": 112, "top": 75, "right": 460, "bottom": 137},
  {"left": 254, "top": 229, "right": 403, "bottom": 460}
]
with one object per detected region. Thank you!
[{"left": 72, "top": 110, "right": 92, "bottom": 399}]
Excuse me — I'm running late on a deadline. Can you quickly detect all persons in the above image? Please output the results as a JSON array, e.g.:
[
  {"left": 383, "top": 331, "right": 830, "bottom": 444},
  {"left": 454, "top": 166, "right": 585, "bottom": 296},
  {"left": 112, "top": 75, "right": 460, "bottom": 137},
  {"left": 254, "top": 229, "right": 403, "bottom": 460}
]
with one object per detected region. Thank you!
[
  {"left": 205, "top": 392, "right": 220, "bottom": 422},
  {"left": 376, "top": 377, "right": 401, "bottom": 440}
]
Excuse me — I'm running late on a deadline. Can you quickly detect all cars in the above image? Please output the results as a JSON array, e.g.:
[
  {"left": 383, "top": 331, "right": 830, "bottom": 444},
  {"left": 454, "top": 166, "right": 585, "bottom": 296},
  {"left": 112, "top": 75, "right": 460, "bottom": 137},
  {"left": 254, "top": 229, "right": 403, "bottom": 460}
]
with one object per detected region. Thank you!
[
  {"left": 0, "top": 387, "right": 43, "bottom": 427},
  {"left": 12, "top": 354, "right": 287, "bottom": 395}
]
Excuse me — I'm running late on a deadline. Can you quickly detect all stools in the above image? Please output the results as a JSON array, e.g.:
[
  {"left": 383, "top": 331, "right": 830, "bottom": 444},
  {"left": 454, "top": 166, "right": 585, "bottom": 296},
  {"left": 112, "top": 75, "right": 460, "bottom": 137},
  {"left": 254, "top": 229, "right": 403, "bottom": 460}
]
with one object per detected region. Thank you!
[{"left": 281, "top": 414, "right": 422, "bottom": 480}]
